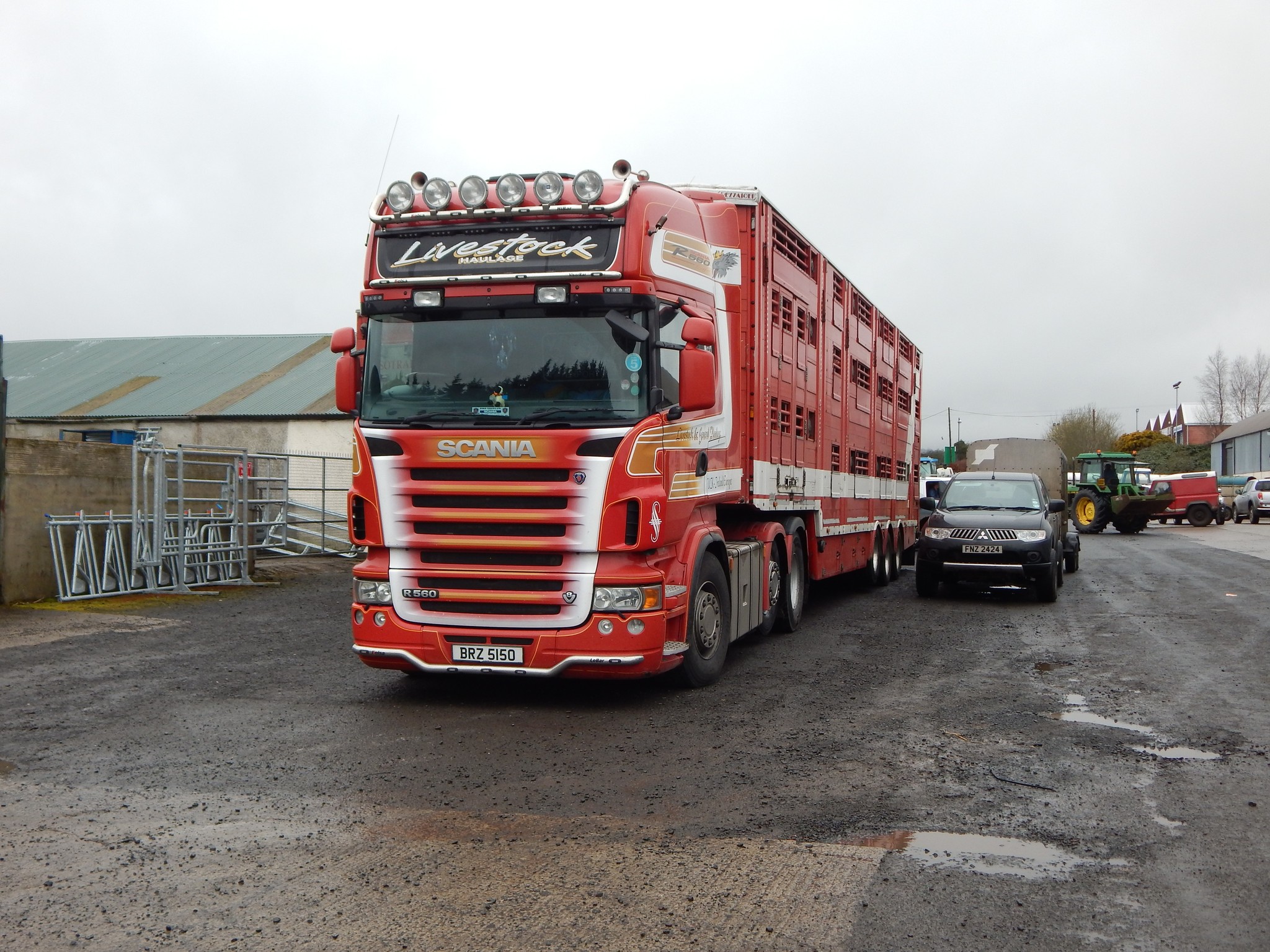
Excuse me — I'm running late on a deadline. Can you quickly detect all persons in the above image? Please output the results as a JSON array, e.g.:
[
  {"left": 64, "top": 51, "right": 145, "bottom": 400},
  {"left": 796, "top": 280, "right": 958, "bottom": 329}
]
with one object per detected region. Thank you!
[
  {"left": 927, "top": 482, "right": 940, "bottom": 499},
  {"left": 1104, "top": 464, "right": 1119, "bottom": 485}
]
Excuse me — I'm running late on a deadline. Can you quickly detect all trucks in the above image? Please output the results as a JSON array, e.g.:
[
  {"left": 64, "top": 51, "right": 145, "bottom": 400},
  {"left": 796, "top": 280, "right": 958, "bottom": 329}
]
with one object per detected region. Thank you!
[{"left": 1216, "top": 475, "right": 1256, "bottom": 521}]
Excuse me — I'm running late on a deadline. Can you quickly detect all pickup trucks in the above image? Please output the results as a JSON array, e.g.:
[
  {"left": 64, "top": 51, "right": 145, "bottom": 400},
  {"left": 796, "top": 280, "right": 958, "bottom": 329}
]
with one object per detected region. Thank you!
[{"left": 1231, "top": 479, "right": 1270, "bottom": 524}]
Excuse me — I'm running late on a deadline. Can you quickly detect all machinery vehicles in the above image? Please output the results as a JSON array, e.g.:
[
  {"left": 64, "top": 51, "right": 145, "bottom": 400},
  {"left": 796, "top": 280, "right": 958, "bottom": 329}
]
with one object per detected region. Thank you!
[{"left": 1067, "top": 450, "right": 1177, "bottom": 535}]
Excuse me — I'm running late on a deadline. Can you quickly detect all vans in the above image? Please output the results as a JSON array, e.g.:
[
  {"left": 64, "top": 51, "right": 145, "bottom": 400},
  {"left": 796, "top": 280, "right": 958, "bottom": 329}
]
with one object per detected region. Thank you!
[{"left": 1147, "top": 471, "right": 1221, "bottom": 527}]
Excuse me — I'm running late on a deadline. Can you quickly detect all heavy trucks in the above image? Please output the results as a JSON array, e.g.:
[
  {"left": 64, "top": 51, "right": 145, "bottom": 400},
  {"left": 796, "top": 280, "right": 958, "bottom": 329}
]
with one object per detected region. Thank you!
[{"left": 330, "top": 158, "right": 922, "bottom": 689}]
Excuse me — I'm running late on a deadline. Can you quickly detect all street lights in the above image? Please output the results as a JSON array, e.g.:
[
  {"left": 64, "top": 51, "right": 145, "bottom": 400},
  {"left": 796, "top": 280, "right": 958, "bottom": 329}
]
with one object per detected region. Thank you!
[
  {"left": 1136, "top": 409, "right": 1139, "bottom": 431},
  {"left": 1173, "top": 381, "right": 1182, "bottom": 445},
  {"left": 957, "top": 417, "right": 962, "bottom": 442}
]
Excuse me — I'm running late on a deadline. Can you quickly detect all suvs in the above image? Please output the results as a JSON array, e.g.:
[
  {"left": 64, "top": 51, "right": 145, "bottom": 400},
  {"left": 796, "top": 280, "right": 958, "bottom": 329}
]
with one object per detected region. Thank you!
[{"left": 916, "top": 455, "right": 1066, "bottom": 603}]
